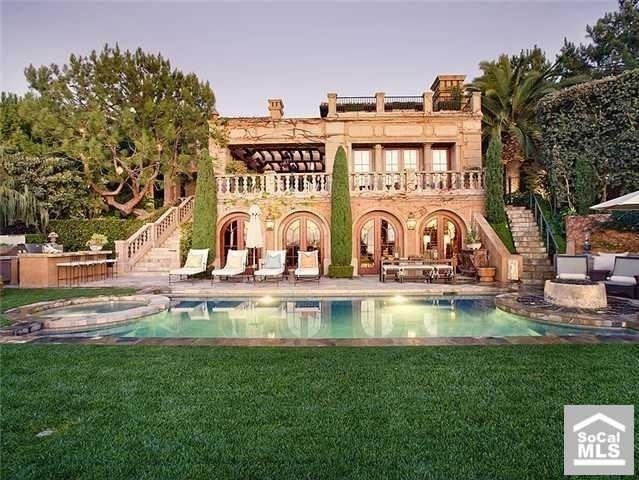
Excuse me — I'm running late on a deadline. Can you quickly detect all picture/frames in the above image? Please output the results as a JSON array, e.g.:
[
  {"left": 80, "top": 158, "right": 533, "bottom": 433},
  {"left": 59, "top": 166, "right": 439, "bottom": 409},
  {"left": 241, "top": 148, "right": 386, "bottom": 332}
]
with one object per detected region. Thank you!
[{"left": 383, "top": 263, "right": 453, "bottom": 283}]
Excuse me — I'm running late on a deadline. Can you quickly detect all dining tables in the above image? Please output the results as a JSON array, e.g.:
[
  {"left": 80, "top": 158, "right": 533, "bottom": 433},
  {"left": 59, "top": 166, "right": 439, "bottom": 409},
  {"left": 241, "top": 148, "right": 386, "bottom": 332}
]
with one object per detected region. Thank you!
[
  {"left": 87, "top": 232, "right": 110, "bottom": 251},
  {"left": 25, "top": 234, "right": 47, "bottom": 253},
  {"left": 460, "top": 227, "right": 482, "bottom": 249}
]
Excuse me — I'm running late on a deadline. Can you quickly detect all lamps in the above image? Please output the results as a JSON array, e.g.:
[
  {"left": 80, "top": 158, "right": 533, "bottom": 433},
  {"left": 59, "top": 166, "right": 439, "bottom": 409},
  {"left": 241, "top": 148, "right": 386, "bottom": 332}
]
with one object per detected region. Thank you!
[
  {"left": 211, "top": 249, "right": 250, "bottom": 282},
  {"left": 553, "top": 252, "right": 590, "bottom": 284},
  {"left": 293, "top": 249, "right": 321, "bottom": 286},
  {"left": 167, "top": 248, "right": 212, "bottom": 286},
  {"left": 257, "top": 249, "right": 287, "bottom": 283},
  {"left": 605, "top": 256, "right": 639, "bottom": 299}
]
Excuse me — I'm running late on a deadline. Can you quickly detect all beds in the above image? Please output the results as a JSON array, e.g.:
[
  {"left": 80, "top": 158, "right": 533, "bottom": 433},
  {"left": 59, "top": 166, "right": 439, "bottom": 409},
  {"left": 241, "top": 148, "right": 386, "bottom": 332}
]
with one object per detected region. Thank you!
[
  {"left": 245, "top": 205, "right": 265, "bottom": 265},
  {"left": 589, "top": 190, "right": 639, "bottom": 210}
]
